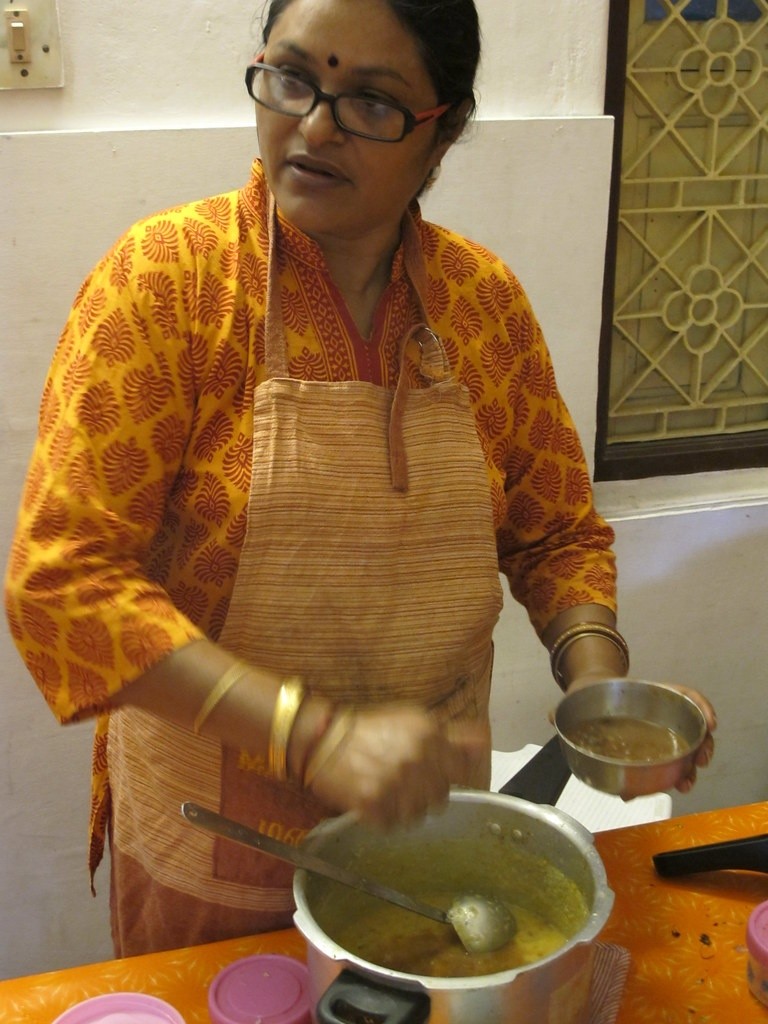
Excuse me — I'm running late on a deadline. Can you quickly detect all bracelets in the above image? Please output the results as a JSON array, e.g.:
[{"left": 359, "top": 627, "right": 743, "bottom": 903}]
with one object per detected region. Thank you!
[
  {"left": 549, "top": 621, "right": 630, "bottom": 690},
  {"left": 301, "top": 704, "right": 355, "bottom": 789},
  {"left": 269, "top": 679, "right": 311, "bottom": 785},
  {"left": 194, "top": 665, "right": 258, "bottom": 734}
]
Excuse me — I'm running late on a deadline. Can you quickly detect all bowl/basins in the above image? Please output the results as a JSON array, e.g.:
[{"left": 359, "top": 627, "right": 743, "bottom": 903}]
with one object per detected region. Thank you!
[
  {"left": 51, "top": 992, "right": 186, "bottom": 1024},
  {"left": 553, "top": 679, "right": 707, "bottom": 796}
]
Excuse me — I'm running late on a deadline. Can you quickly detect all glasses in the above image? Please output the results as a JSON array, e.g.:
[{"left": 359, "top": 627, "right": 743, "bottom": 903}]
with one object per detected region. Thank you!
[{"left": 244, "top": 47, "right": 458, "bottom": 144}]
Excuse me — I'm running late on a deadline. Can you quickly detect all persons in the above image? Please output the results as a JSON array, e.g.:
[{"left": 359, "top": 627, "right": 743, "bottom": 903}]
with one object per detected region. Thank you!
[{"left": 3, "top": 0, "right": 718, "bottom": 962}]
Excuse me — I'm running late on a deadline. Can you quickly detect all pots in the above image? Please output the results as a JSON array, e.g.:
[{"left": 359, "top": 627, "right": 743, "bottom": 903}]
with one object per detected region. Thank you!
[{"left": 292, "top": 734, "right": 615, "bottom": 1024}]
